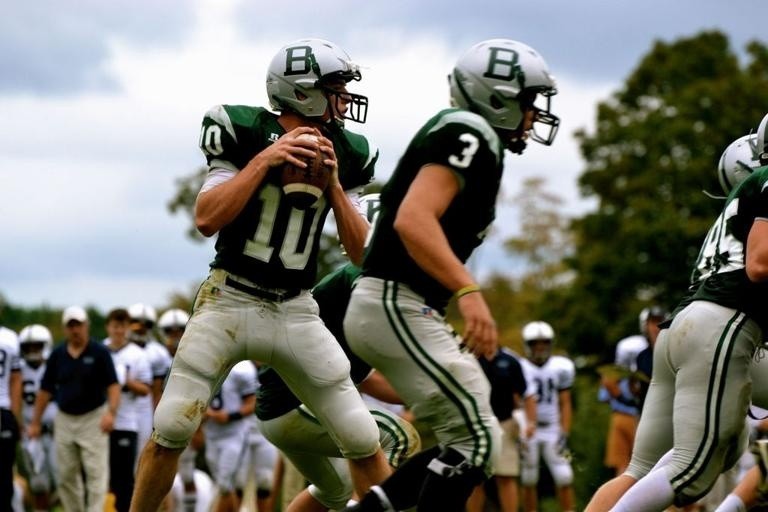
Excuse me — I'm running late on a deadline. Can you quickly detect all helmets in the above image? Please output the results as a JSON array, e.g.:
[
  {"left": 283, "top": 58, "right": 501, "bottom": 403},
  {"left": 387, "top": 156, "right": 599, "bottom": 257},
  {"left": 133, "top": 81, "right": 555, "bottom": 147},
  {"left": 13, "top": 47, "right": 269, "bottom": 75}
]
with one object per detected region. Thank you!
[
  {"left": 267, "top": 37, "right": 369, "bottom": 125},
  {"left": 718, "top": 116, "right": 763, "bottom": 198},
  {"left": 17, "top": 324, "right": 53, "bottom": 360},
  {"left": 156, "top": 307, "right": 190, "bottom": 348},
  {"left": 448, "top": 38, "right": 560, "bottom": 155},
  {"left": 522, "top": 320, "right": 558, "bottom": 360},
  {"left": 127, "top": 302, "right": 158, "bottom": 345}
]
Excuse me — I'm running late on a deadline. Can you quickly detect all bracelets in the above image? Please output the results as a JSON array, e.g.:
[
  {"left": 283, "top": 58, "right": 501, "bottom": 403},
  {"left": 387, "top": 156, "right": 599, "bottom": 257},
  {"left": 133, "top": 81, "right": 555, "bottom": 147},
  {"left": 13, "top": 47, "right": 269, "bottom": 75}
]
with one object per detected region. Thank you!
[{"left": 453, "top": 284, "right": 482, "bottom": 299}]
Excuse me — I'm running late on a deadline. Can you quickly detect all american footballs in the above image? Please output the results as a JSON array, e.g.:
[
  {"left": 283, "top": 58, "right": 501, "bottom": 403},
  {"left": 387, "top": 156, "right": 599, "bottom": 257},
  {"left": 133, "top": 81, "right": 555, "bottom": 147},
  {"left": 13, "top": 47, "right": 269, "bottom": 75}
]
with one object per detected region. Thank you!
[{"left": 281, "top": 129, "right": 332, "bottom": 210}]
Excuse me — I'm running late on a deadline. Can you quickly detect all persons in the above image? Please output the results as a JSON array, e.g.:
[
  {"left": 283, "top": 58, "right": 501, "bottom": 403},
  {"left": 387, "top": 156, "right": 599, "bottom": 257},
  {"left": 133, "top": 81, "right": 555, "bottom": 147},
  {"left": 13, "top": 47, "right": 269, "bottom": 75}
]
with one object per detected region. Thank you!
[
  {"left": 0, "top": 303, "right": 282, "bottom": 511},
  {"left": 583, "top": 117, "right": 768, "bottom": 512},
  {"left": 347, "top": 37, "right": 563, "bottom": 511},
  {"left": 257, "top": 194, "right": 422, "bottom": 512},
  {"left": 127, "top": 33, "right": 389, "bottom": 511},
  {"left": 468, "top": 322, "right": 574, "bottom": 511}
]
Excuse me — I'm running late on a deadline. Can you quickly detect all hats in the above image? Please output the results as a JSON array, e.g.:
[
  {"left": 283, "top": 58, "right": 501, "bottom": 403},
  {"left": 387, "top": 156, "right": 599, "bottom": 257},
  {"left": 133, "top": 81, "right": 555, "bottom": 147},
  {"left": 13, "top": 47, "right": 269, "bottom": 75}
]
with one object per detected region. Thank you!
[{"left": 59, "top": 305, "right": 89, "bottom": 325}]
